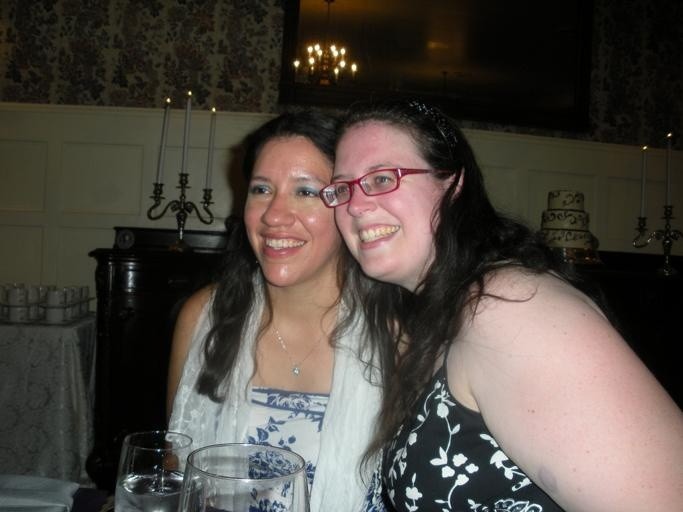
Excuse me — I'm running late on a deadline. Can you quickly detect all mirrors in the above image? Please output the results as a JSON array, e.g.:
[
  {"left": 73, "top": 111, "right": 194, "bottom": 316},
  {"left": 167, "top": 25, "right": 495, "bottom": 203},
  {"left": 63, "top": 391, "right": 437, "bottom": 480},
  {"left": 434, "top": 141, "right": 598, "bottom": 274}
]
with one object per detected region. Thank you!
[{"left": 276, "top": 0, "right": 592, "bottom": 129}]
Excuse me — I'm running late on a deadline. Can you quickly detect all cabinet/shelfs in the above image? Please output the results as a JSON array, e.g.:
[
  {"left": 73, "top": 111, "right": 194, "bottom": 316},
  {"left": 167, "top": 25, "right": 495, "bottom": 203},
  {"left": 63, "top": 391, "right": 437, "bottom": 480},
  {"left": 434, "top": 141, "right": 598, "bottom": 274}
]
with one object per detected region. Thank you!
[{"left": 88, "top": 226, "right": 683, "bottom": 512}]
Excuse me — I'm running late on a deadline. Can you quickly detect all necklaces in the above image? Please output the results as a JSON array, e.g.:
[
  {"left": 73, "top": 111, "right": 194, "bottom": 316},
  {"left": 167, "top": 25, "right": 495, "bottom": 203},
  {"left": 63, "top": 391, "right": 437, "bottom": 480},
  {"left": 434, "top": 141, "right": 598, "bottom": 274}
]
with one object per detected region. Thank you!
[{"left": 270, "top": 314, "right": 338, "bottom": 376}]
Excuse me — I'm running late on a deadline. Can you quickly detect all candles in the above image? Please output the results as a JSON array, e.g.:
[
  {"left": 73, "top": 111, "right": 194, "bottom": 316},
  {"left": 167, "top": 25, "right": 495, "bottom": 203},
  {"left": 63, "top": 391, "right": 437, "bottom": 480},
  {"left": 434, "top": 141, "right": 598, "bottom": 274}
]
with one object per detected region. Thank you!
[
  {"left": 181, "top": 91, "right": 192, "bottom": 174},
  {"left": 157, "top": 98, "right": 171, "bottom": 184},
  {"left": 640, "top": 146, "right": 647, "bottom": 218},
  {"left": 206, "top": 107, "right": 215, "bottom": 189},
  {"left": 665, "top": 133, "right": 672, "bottom": 205}
]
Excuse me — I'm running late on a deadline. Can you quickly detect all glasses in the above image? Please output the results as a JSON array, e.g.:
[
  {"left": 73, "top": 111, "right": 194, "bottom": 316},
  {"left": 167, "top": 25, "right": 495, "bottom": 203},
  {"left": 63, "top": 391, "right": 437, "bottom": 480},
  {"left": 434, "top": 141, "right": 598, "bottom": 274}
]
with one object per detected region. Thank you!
[{"left": 319, "top": 168, "right": 447, "bottom": 208}]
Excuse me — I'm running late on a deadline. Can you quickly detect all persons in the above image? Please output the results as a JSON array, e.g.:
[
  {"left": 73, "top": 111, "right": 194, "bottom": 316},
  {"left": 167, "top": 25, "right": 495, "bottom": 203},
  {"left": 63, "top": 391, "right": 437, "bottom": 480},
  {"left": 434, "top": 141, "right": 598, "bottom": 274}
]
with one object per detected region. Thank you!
[
  {"left": 166, "top": 106, "right": 409, "bottom": 511},
  {"left": 334, "top": 96, "right": 683, "bottom": 511}
]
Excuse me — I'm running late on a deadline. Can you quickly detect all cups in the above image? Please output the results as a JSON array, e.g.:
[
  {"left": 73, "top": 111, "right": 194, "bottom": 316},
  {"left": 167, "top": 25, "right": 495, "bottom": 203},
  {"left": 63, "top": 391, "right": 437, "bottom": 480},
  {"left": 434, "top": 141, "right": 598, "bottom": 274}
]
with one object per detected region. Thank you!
[
  {"left": 177, "top": 442, "right": 310, "bottom": 512},
  {"left": 3, "top": 306, "right": 92, "bottom": 325},
  {"left": 112, "top": 430, "right": 197, "bottom": 512},
  {"left": 0, "top": 279, "right": 89, "bottom": 305}
]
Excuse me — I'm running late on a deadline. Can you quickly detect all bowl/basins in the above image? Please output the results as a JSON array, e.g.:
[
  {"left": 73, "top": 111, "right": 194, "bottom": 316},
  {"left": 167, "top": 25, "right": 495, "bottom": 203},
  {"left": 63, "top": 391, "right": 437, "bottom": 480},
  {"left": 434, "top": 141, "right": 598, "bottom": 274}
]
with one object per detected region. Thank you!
[{"left": 181, "top": 229, "right": 227, "bottom": 255}]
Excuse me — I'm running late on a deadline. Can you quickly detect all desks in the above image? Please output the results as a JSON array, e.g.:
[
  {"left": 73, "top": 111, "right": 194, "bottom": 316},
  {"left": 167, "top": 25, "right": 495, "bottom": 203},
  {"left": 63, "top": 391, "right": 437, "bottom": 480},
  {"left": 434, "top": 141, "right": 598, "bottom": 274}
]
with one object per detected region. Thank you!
[{"left": 0, "top": 314, "right": 95, "bottom": 481}]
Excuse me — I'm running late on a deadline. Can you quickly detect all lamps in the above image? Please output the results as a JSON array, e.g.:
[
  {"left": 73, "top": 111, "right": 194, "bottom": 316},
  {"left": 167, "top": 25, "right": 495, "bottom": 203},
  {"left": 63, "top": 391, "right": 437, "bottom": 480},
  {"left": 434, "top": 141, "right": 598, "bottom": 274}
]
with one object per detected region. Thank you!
[{"left": 294, "top": 1, "right": 358, "bottom": 87}]
